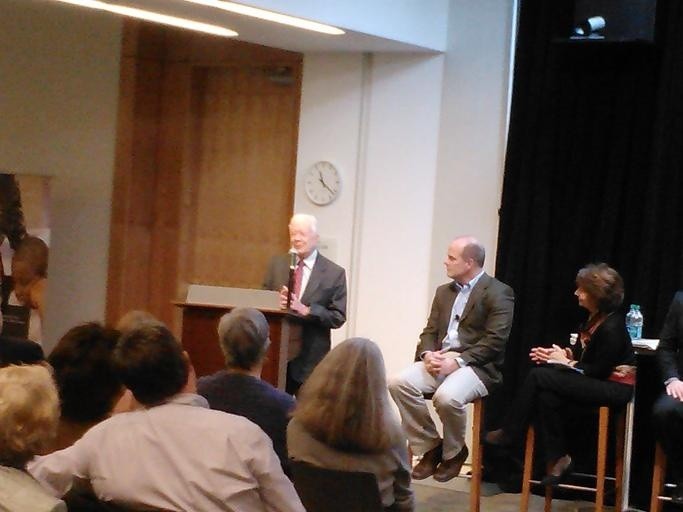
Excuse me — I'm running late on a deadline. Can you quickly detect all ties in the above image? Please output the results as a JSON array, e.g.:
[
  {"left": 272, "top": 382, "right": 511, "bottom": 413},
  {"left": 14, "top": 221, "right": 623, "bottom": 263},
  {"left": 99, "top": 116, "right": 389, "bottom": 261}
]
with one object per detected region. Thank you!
[{"left": 293, "top": 260, "right": 304, "bottom": 307}]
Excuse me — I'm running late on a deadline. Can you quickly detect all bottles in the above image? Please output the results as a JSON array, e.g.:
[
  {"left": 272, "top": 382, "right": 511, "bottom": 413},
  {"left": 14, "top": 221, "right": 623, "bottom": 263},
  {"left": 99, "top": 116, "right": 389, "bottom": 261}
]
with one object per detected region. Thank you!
[
  {"left": 630, "top": 306, "right": 643, "bottom": 340},
  {"left": 626, "top": 305, "right": 636, "bottom": 334}
]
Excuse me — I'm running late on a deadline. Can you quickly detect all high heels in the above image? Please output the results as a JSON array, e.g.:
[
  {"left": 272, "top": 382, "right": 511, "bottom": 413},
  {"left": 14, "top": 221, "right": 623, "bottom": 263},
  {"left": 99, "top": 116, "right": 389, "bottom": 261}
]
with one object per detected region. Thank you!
[
  {"left": 479, "top": 431, "right": 510, "bottom": 450},
  {"left": 540, "top": 458, "right": 573, "bottom": 486}
]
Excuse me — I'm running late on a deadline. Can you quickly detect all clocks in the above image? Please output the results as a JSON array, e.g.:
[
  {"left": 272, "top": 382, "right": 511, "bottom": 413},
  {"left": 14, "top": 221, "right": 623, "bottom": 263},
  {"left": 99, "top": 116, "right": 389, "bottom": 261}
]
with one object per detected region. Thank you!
[{"left": 306, "top": 160, "right": 341, "bottom": 206}]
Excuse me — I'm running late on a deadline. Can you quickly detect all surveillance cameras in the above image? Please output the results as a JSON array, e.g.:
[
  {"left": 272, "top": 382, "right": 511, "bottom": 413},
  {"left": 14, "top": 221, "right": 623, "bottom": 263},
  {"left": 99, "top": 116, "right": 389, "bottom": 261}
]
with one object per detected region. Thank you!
[{"left": 571, "top": 16, "right": 606, "bottom": 40}]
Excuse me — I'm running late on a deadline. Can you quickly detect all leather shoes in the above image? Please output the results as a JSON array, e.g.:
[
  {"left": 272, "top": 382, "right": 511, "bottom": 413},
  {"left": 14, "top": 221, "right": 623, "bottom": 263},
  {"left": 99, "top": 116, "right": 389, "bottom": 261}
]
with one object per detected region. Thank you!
[
  {"left": 411, "top": 445, "right": 443, "bottom": 479},
  {"left": 433, "top": 445, "right": 469, "bottom": 482},
  {"left": 673, "top": 481, "right": 683, "bottom": 504}
]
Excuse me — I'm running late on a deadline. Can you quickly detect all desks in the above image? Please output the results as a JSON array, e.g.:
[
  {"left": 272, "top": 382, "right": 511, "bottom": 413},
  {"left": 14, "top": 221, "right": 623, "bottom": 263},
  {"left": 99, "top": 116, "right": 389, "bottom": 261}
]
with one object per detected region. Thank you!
[{"left": 171, "top": 303, "right": 300, "bottom": 391}]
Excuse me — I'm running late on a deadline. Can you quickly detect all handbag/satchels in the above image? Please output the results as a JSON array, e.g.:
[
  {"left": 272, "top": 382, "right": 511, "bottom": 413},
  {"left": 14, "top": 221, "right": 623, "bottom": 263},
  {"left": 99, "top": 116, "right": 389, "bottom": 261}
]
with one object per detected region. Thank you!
[{"left": 607, "top": 365, "right": 638, "bottom": 385}]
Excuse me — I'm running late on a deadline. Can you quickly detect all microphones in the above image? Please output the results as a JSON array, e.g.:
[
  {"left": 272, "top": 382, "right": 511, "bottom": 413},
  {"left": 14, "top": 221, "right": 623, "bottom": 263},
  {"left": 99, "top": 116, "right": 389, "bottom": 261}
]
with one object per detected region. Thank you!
[{"left": 287, "top": 248, "right": 299, "bottom": 307}]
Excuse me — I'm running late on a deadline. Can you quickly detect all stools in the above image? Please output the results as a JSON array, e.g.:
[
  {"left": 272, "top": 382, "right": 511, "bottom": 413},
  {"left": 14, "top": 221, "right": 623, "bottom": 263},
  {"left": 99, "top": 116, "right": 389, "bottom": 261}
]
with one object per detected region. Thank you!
[
  {"left": 408, "top": 393, "right": 483, "bottom": 512},
  {"left": 520, "top": 406, "right": 627, "bottom": 512}
]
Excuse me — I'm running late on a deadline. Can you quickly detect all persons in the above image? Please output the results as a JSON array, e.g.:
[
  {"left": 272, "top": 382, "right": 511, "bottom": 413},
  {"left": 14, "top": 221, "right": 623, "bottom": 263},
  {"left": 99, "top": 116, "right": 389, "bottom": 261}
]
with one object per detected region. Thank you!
[
  {"left": 266, "top": 216, "right": 345, "bottom": 392},
  {"left": 195, "top": 308, "right": 296, "bottom": 437},
  {"left": 483, "top": 266, "right": 636, "bottom": 487},
  {"left": 7, "top": 237, "right": 48, "bottom": 345},
  {"left": 25, "top": 325, "right": 306, "bottom": 512},
  {"left": 0, "top": 364, "right": 66, "bottom": 511},
  {"left": 1, "top": 338, "right": 45, "bottom": 364},
  {"left": 288, "top": 339, "right": 415, "bottom": 511},
  {"left": 653, "top": 290, "right": 683, "bottom": 502},
  {"left": 0, "top": 173, "right": 28, "bottom": 281},
  {"left": 111, "top": 312, "right": 162, "bottom": 415},
  {"left": 389, "top": 238, "right": 515, "bottom": 481},
  {"left": 43, "top": 325, "right": 124, "bottom": 448}
]
